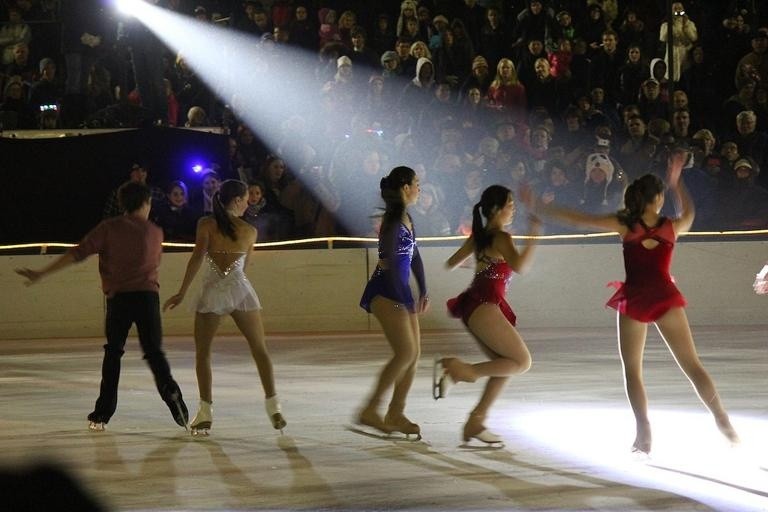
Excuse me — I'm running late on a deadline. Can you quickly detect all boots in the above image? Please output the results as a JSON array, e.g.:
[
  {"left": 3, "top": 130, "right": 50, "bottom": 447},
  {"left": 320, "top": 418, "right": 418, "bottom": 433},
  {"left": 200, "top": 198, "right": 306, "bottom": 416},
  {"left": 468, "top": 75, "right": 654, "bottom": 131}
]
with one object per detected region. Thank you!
[
  {"left": 383, "top": 400, "right": 424, "bottom": 435},
  {"left": 190, "top": 396, "right": 213, "bottom": 432},
  {"left": 631, "top": 417, "right": 653, "bottom": 454},
  {"left": 440, "top": 356, "right": 473, "bottom": 400},
  {"left": 263, "top": 392, "right": 287, "bottom": 430},
  {"left": 359, "top": 394, "right": 399, "bottom": 433},
  {"left": 160, "top": 378, "right": 189, "bottom": 429},
  {"left": 713, "top": 411, "right": 740, "bottom": 444},
  {"left": 87, "top": 410, "right": 109, "bottom": 425},
  {"left": 463, "top": 409, "right": 504, "bottom": 445}
]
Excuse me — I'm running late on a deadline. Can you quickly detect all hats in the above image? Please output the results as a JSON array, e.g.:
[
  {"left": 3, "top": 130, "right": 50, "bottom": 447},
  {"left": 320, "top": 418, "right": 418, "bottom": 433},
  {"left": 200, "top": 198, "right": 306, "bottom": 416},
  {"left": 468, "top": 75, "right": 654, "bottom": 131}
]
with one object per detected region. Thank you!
[
  {"left": 585, "top": 153, "right": 613, "bottom": 183},
  {"left": 336, "top": 51, "right": 400, "bottom": 70}
]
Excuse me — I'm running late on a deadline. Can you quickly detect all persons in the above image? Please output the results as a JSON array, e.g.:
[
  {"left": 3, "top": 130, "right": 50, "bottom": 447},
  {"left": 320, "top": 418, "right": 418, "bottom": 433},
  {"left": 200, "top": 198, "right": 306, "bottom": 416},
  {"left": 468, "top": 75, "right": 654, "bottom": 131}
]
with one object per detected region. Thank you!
[
  {"left": 1, "top": 1, "right": 768, "bottom": 253},
  {"left": 431, "top": 186, "right": 544, "bottom": 452},
  {"left": 161, "top": 180, "right": 288, "bottom": 433},
  {"left": 356, "top": 166, "right": 429, "bottom": 436},
  {"left": 514, "top": 145, "right": 746, "bottom": 455},
  {"left": 13, "top": 180, "right": 190, "bottom": 431}
]
